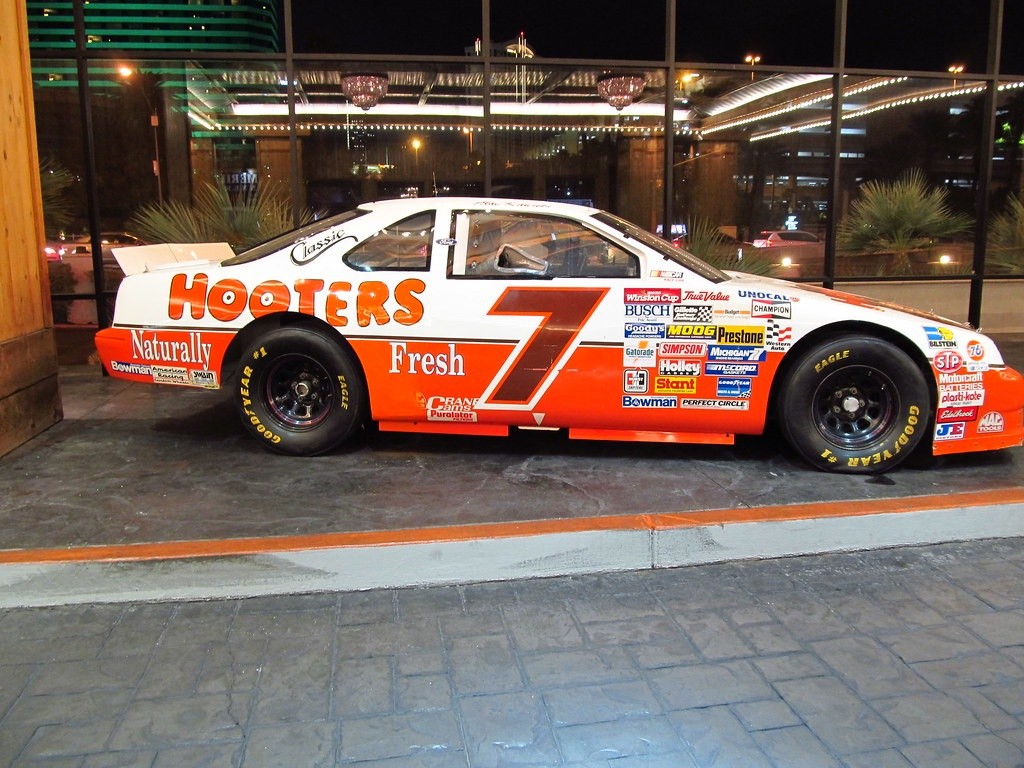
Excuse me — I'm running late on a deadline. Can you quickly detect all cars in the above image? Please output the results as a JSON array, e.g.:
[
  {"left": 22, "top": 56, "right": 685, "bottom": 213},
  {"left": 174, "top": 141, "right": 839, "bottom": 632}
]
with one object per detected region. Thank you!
[
  {"left": 43, "top": 230, "right": 149, "bottom": 280},
  {"left": 669, "top": 230, "right": 823, "bottom": 253},
  {"left": 94, "top": 196, "right": 1023, "bottom": 476}
]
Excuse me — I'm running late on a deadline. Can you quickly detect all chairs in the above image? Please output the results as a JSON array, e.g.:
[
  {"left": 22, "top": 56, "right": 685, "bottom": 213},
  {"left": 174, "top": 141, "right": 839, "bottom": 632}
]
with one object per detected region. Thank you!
[{"left": 466, "top": 220, "right": 513, "bottom": 275}]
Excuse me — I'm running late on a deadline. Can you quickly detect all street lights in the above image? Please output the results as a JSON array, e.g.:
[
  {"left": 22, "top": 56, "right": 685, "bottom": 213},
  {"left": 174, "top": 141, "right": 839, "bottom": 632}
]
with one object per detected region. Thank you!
[
  {"left": 745, "top": 55, "right": 761, "bottom": 80},
  {"left": 949, "top": 66, "right": 964, "bottom": 90}
]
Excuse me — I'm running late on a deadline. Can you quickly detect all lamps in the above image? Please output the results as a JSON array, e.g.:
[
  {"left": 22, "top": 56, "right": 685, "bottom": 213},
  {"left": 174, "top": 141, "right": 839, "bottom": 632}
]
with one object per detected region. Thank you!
[
  {"left": 596, "top": 74, "right": 644, "bottom": 110},
  {"left": 341, "top": 73, "right": 388, "bottom": 110}
]
